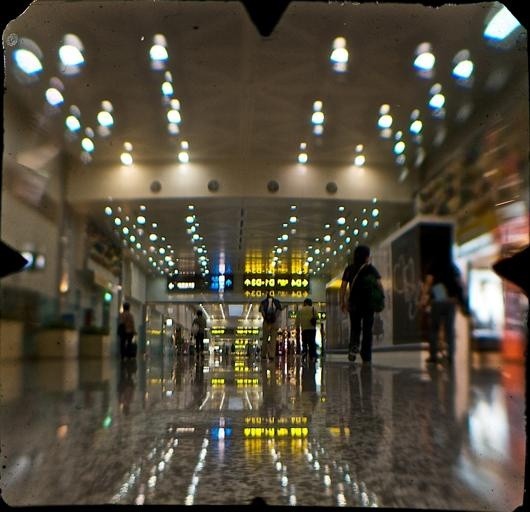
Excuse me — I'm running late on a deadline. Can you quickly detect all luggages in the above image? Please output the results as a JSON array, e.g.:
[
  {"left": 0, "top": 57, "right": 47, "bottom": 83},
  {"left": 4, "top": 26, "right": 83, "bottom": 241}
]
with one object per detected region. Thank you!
[{"left": 127, "top": 334, "right": 137, "bottom": 358}]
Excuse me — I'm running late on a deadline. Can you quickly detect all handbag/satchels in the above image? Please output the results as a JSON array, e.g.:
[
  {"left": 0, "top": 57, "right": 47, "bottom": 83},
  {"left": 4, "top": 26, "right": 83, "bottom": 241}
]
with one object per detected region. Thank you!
[
  {"left": 364, "top": 273, "right": 384, "bottom": 313},
  {"left": 191, "top": 323, "right": 200, "bottom": 336},
  {"left": 309, "top": 315, "right": 317, "bottom": 327}
]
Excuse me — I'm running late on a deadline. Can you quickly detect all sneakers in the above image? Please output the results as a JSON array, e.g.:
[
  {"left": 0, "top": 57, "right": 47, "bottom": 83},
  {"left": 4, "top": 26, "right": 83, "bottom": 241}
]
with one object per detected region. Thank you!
[{"left": 348, "top": 344, "right": 359, "bottom": 361}]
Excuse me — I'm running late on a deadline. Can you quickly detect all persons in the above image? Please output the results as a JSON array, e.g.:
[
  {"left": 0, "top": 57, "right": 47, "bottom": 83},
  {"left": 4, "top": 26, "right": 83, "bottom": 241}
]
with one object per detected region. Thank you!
[
  {"left": 247, "top": 291, "right": 319, "bottom": 361},
  {"left": 420, "top": 246, "right": 474, "bottom": 363},
  {"left": 339, "top": 245, "right": 382, "bottom": 362},
  {"left": 120, "top": 302, "right": 137, "bottom": 362},
  {"left": 191, "top": 309, "right": 207, "bottom": 353}
]
